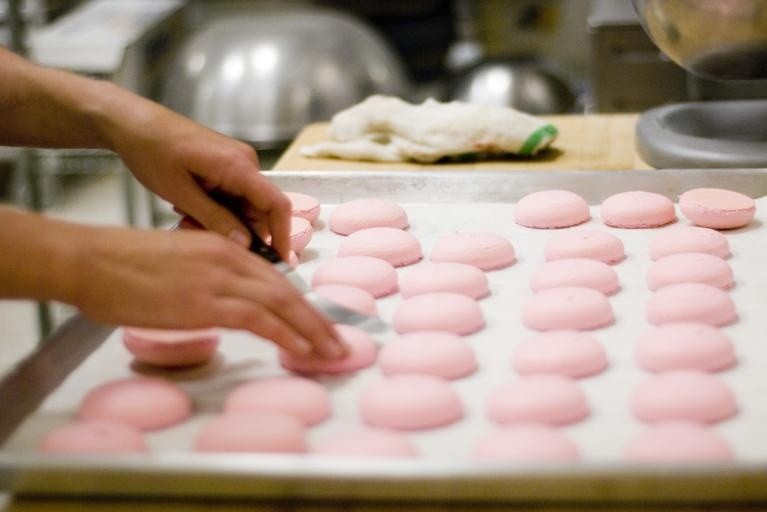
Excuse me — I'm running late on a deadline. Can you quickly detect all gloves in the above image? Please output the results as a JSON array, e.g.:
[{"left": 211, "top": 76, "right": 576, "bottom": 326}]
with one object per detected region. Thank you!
[{"left": 301, "top": 93, "right": 559, "bottom": 167}]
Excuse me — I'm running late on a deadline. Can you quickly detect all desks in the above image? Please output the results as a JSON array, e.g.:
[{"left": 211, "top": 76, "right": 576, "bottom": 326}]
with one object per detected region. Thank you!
[{"left": 277, "top": 113, "right": 649, "bottom": 170}]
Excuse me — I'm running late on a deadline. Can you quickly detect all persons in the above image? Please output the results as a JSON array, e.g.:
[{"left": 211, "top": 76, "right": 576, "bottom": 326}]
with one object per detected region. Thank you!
[{"left": 0, "top": 46, "right": 349, "bottom": 361}]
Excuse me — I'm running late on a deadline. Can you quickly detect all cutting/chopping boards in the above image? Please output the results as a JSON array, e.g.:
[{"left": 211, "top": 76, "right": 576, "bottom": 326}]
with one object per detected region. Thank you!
[{"left": 1, "top": 112, "right": 767, "bottom": 502}]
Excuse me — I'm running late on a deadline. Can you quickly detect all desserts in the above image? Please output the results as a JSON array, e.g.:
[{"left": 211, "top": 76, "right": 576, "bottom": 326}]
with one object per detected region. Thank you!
[{"left": 34, "top": 186, "right": 755, "bottom": 471}]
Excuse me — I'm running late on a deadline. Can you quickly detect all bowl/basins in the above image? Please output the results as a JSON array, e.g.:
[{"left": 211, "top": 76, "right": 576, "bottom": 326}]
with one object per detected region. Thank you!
[
  {"left": 629, "top": 0, "right": 766, "bottom": 80},
  {"left": 630, "top": 98, "right": 763, "bottom": 167},
  {"left": 155, "top": 4, "right": 421, "bottom": 150},
  {"left": 453, "top": 64, "right": 587, "bottom": 116}
]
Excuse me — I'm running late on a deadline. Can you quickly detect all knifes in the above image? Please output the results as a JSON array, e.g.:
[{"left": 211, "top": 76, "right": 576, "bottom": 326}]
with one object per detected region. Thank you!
[{"left": 181, "top": 189, "right": 392, "bottom": 341}]
